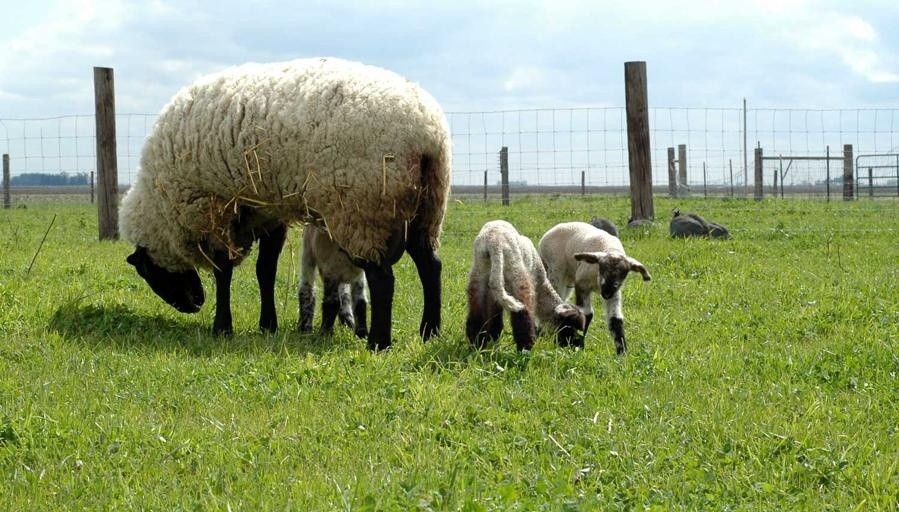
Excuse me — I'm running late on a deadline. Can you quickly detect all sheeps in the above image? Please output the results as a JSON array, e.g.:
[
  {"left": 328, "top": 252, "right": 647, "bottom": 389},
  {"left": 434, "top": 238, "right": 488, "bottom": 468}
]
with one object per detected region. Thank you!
[
  {"left": 464, "top": 219, "right": 587, "bottom": 351},
  {"left": 538, "top": 220, "right": 653, "bottom": 354},
  {"left": 115, "top": 55, "right": 454, "bottom": 353},
  {"left": 670, "top": 209, "right": 733, "bottom": 242},
  {"left": 295, "top": 221, "right": 370, "bottom": 340},
  {"left": 592, "top": 216, "right": 654, "bottom": 238}
]
